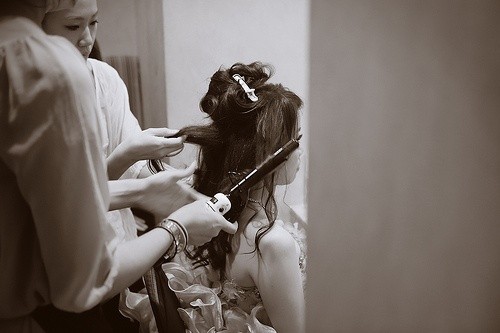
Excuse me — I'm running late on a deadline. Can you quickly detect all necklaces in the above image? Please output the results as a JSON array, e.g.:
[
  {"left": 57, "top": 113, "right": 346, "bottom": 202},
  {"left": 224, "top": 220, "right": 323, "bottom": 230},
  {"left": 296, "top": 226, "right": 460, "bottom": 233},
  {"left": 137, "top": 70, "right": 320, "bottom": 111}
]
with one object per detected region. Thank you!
[{"left": 247, "top": 197, "right": 276, "bottom": 216}]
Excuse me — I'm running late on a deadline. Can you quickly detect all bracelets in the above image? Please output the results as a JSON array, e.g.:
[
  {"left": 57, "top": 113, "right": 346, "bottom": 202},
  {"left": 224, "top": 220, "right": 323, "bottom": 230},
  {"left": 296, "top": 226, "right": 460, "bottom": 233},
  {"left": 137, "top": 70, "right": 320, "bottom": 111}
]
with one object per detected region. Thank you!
[
  {"left": 154, "top": 219, "right": 181, "bottom": 262},
  {"left": 166, "top": 217, "right": 187, "bottom": 254}
]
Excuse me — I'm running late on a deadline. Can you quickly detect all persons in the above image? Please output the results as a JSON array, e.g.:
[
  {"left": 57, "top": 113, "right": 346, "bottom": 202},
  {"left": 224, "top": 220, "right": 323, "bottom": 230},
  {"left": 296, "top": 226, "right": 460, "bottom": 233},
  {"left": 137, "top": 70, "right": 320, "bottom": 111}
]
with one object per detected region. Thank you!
[
  {"left": 0, "top": 0, "right": 238, "bottom": 333},
  {"left": 41, "top": 0, "right": 196, "bottom": 333},
  {"left": 146, "top": 62, "right": 305, "bottom": 333}
]
